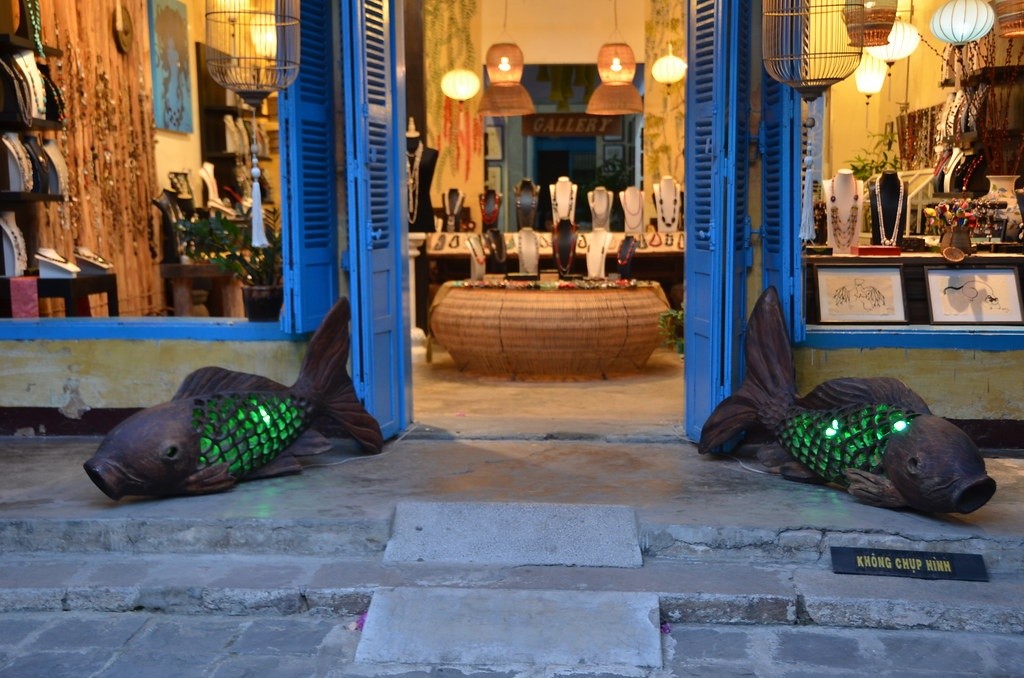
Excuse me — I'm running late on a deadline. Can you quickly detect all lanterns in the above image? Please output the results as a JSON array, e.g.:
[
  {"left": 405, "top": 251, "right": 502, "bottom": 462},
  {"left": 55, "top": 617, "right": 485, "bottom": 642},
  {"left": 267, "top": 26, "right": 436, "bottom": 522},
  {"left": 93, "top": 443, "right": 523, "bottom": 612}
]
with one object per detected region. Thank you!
[
  {"left": 930, "top": 0, "right": 1024, "bottom": 64},
  {"left": 652, "top": 45, "right": 687, "bottom": 98},
  {"left": 441, "top": 69, "right": 481, "bottom": 109},
  {"left": 844, "top": 0, "right": 920, "bottom": 126}
]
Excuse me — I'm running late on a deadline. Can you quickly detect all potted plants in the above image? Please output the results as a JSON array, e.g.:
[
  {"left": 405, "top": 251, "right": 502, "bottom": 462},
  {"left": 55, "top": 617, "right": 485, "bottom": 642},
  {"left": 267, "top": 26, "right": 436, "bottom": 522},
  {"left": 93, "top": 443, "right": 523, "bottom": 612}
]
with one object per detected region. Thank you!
[{"left": 170, "top": 202, "right": 284, "bottom": 323}]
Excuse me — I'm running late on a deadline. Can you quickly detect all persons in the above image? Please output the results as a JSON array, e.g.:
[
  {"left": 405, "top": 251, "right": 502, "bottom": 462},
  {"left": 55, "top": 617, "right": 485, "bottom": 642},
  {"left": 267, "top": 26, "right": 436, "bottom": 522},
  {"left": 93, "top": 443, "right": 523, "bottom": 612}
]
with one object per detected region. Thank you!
[{"left": 156, "top": 7, "right": 192, "bottom": 133}]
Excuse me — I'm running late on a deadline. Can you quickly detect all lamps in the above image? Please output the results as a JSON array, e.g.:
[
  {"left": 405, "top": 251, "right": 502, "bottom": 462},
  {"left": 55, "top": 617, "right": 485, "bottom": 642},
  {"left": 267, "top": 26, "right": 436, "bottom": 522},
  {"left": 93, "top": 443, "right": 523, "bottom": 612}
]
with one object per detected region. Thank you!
[
  {"left": 842, "top": 0, "right": 1023, "bottom": 110},
  {"left": 440, "top": 0, "right": 686, "bottom": 114}
]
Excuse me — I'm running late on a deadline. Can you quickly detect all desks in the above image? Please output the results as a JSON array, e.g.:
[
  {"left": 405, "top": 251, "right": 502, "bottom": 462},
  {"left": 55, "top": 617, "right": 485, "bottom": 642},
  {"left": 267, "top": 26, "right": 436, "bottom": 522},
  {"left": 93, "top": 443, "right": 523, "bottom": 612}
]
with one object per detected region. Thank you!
[{"left": 160, "top": 260, "right": 247, "bottom": 319}]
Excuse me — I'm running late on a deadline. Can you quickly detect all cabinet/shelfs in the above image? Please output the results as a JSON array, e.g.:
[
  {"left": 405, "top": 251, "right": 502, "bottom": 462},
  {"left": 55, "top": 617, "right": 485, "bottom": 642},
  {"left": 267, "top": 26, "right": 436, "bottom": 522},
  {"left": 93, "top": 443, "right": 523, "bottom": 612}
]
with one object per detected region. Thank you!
[{"left": 0, "top": 33, "right": 120, "bottom": 318}]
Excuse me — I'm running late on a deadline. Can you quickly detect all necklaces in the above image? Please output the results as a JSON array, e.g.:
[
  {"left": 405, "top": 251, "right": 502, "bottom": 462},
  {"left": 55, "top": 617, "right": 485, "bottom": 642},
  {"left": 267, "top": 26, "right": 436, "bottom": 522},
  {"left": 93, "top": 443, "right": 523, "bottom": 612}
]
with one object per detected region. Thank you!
[
  {"left": 0, "top": 0, "right": 159, "bottom": 272},
  {"left": 404, "top": 143, "right": 423, "bottom": 225},
  {"left": 902, "top": 102, "right": 980, "bottom": 191},
  {"left": 442, "top": 182, "right": 679, "bottom": 279},
  {"left": 927, "top": 200, "right": 1000, "bottom": 241},
  {"left": 830, "top": 177, "right": 904, "bottom": 252}
]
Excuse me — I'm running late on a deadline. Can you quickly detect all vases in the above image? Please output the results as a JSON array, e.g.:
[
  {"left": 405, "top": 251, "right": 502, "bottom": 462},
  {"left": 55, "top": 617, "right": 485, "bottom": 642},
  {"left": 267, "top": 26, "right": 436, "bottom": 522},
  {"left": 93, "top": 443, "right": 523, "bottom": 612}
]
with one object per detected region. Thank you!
[{"left": 985, "top": 175, "right": 1022, "bottom": 238}]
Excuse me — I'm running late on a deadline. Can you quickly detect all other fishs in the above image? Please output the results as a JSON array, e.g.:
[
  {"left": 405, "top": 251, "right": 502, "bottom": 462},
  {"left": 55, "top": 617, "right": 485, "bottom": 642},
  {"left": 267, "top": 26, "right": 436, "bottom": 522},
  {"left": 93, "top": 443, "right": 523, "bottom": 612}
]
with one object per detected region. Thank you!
[
  {"left": 698, "top": 284, "right": 997, "bottom": 514},
  {"left": 83, "top": 298, "right": 383, "bottom": 501}
]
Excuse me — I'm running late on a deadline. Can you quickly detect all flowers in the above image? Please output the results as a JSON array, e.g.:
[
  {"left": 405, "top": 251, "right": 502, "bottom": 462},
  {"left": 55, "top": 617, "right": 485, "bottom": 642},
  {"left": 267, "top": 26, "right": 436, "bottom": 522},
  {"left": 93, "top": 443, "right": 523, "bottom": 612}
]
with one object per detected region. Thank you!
[{"left": 917, "top": 27, "right": 1024, "bottom": 177}]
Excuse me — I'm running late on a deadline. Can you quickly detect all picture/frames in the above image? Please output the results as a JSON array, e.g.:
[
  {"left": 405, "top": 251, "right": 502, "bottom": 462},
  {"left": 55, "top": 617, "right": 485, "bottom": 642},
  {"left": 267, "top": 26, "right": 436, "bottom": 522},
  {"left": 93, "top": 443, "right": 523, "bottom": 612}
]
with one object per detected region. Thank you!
[
  {"left": 485, "top": 124, "right": 503, "bottom": 194},
  {"left": 924, "top": 265, "right": 1024, "bottom": 326},
  {"left": 812, "top": 262, "right": 911, "bottom": 326},
  {"left": 604, "top": 115, "right": 626, "bottom": 174}
]
